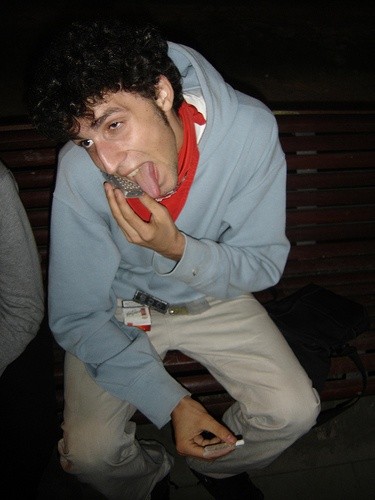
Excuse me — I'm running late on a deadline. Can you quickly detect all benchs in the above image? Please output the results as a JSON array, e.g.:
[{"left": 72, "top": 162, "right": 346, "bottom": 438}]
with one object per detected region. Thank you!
[{"left": 0, "top": 103, "right": 375, "bottom": 425}]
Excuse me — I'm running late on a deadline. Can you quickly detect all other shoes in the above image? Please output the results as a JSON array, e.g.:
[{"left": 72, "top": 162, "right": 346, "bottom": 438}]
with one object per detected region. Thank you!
[
  {"left": 150, "top": 473, "right": 180, "bottom": 500},
  {"left": 188, "top": 466, "right": 264, "bottom": 500}
]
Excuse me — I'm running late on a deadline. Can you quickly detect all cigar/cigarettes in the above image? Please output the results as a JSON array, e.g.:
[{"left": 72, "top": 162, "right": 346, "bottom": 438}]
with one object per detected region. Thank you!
[{"left": 202, "top": 439, "right": 245, "bottom": 454}]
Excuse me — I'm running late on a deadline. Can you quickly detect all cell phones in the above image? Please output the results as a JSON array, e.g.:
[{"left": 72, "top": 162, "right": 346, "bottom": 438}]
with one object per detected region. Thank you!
[{"left": 99, "top": 170, "right": 143, "bottom": 198}]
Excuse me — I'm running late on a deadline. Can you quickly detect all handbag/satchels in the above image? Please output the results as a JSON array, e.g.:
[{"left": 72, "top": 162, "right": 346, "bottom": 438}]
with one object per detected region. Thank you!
[{"left": 265, "top": 282, "right": 371, "bottom": 358}]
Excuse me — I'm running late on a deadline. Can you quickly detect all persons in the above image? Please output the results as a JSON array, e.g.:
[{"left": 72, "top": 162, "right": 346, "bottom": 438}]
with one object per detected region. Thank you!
[
  {"left": 0, "top": 159, "right": 45, "bottom": 379},
  {"left": 22, "top": 13, "right": 322, "bottom": 500}
]
